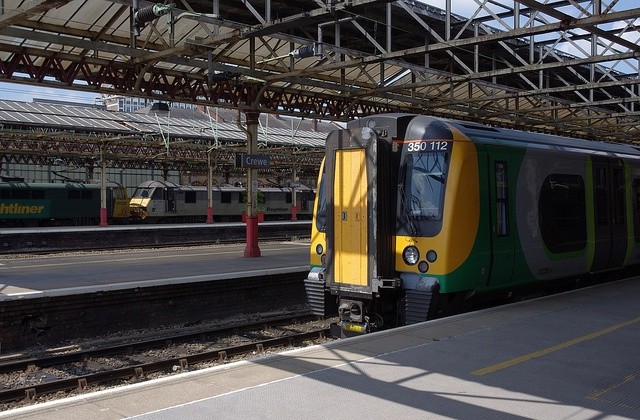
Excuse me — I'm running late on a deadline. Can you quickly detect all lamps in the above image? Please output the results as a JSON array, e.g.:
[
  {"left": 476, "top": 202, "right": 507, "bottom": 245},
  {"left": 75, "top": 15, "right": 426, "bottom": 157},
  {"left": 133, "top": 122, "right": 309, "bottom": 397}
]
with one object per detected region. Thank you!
[{"left": 149, "top": 99, "right": 170, "bottom": 112}]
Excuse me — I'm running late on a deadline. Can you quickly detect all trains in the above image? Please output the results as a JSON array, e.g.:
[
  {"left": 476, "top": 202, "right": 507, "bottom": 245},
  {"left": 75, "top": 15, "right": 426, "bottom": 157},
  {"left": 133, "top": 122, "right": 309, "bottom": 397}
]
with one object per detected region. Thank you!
[
  {"left": 1, "top": 174, "right": 114, "bottom": 226},
  {"left": 127, "top": 181, "right": 318, "bottom": 229},
  {"left": 301, "top": 112, "right": 640, "bottom": 337}
]
